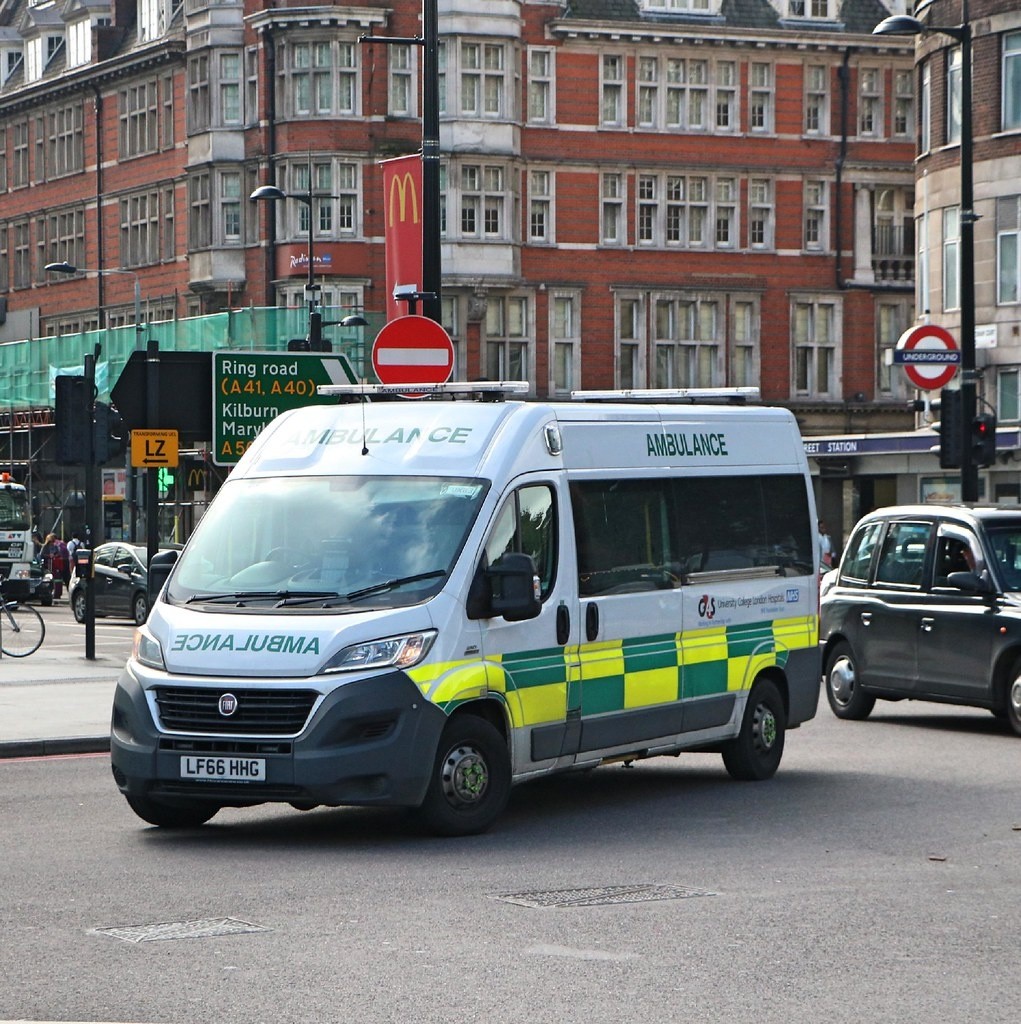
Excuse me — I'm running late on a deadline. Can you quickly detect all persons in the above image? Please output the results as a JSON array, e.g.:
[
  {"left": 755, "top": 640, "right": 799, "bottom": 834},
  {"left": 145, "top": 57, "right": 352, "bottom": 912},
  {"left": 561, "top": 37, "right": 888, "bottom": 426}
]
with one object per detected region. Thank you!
[
  {"left": 33, "top": 532, "right": 86, "bottom": 586},
  {"left": 818, "top": 520, "right": 831, "bottom": 564}
]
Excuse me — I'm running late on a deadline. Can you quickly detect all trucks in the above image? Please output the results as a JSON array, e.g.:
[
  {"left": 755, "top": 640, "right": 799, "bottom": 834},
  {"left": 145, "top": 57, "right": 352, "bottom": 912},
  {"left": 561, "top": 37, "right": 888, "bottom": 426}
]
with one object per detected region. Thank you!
[{"left": 0, "top": 473, "right": 35, "bottom": 582}]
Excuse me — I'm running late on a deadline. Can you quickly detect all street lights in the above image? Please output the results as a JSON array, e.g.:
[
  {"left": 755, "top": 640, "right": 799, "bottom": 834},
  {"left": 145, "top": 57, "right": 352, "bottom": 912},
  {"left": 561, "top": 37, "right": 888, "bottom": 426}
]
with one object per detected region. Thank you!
[
  {"left": 395, "top": 292, "right": 441, "bottom": 399},
  {"left": 870, "top": 13, "right": 981, "bottom": 503},
  {"left": 249, "top": 184, "right": 370, "bottom": 351},
  {"left": 44, "top": 263, "right": 158, "bottom": 623}
]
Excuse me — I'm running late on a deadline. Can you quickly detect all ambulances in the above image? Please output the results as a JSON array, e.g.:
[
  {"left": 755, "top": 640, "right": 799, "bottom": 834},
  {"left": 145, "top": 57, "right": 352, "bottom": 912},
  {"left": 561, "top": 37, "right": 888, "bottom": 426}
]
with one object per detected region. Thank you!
[{"left": 108, "top": 381, "right": 827, "bottom": 837}]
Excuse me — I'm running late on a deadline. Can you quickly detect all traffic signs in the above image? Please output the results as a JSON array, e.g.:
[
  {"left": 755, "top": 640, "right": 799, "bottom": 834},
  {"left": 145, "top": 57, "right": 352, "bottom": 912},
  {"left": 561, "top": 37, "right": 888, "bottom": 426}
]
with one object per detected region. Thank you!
[{"left": 132, "top": 429, "right": 181, "bottom": 468}]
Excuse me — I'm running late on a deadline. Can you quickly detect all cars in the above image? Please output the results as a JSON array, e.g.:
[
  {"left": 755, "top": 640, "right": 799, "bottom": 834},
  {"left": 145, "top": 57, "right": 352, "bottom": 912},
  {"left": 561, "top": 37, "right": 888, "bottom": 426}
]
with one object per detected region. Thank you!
[
  {"left": 817, "top": 501, "right": 1021, "bottom": 736},
  {"left": 69, "top": 540, "right": 188, "bottom": 625},
  {"left": 14, "top": 552, "right": 54, "bottom": 606}
]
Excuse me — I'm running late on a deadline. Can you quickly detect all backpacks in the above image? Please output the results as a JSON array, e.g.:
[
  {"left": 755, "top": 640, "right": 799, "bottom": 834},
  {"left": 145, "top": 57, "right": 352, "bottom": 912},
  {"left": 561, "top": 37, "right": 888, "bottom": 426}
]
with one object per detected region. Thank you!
[{"left": 72, "top": 540, "right": 82, "bottom": 561}]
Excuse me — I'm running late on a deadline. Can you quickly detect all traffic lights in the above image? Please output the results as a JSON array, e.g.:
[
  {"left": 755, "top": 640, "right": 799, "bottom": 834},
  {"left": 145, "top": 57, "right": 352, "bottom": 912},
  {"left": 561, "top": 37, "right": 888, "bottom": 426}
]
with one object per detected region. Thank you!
[
  {"left": 972, "top": 413, "right": 996, "bottom": 467},
  {"left": 930, "top": 388, "right": 960, "bottom": 470}
]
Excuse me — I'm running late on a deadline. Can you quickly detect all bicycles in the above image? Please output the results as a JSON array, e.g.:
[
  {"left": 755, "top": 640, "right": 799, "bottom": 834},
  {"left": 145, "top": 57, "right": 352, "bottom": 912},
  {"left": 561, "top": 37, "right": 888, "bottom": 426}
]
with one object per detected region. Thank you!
[{"left": 0, "top": 574, "right": 46, "bottom": 659}]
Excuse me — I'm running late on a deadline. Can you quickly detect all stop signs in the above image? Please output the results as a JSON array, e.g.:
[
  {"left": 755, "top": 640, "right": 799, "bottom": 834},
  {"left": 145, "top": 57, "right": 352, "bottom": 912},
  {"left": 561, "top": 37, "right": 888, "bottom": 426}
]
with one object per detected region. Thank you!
[{"left": 371, "top": 314, "right": 458, "bottom": 398}]
